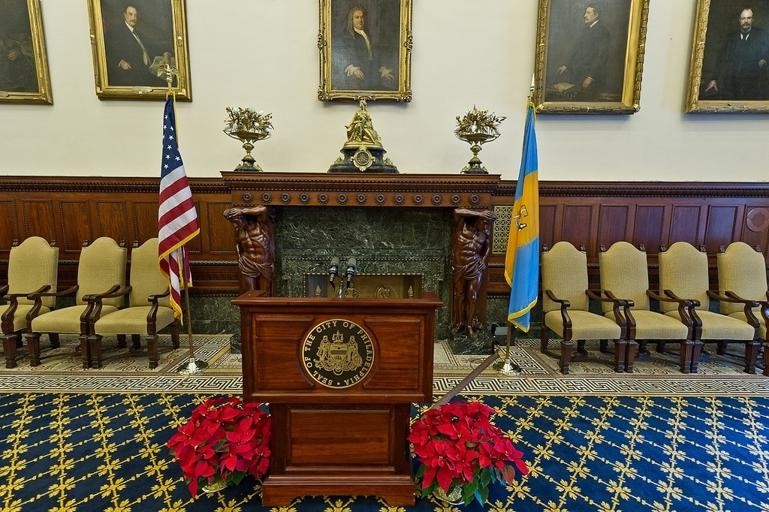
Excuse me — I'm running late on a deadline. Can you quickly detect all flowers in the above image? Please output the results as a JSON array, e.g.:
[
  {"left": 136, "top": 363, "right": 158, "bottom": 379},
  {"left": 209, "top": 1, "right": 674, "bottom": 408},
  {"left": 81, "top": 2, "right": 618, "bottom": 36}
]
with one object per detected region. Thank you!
[
  {"left": 169, "top": 396, "right": 273, "bottom": 497},
  {"left": 407, "top": 402, "right": 528, "bottom": 506}
]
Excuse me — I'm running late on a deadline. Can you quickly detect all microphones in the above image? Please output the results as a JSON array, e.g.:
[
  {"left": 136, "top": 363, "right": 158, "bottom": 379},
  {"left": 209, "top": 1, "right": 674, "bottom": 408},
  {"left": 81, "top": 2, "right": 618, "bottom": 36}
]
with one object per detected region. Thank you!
[
  {"left": 329, "top": 256, "right": 340, "bottom": 282},
  {"left": 346, "top": 257, "right": 357, "bottom": 282}
]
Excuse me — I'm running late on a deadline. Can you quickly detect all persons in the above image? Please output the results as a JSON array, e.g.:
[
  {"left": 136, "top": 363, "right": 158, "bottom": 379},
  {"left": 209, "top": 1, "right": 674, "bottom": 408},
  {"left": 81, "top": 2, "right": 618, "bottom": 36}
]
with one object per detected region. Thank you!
[
  {"left": 343, "top": 98, "right": 381, "bottom": 144},
  {"left": 105, "top": 4, "right": 176, "bottom": 89},
  {"left": 705, "top": 5, "right": 768, "bottom": 99},
  {"left": 554, "top": 4, "right": 611, "bottom": 98},
  {"left": 334, "top": 6, "right": 396, "bottom": 90},
  {"left": 0, "top": 32, "right": 29, "bottom": 89}
]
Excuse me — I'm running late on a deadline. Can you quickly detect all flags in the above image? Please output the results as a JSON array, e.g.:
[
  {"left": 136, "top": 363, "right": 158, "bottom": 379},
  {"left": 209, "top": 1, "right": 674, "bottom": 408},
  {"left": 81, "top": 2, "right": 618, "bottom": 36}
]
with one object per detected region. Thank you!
[
  {"left": 504, "top": 102, "right": 540, "bottom": 334},
  {"left": 157, "top": 92, "right": 202, "bottom": 327}
]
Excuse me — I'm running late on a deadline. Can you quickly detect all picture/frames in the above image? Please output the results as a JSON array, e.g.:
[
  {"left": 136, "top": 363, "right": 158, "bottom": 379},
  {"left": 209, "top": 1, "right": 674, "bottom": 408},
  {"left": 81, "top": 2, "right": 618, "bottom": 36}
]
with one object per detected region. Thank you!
[
  {"left": 685, "top": 0, "right": 769, "bottom": 113},
  {"left": 318, "top": 0, "right": 412, "bottom": 103},
  {"left": 0, "top": 0, "right": 53, "bottom": 105},
  {"left": 86, "top": 0, "right": 191, "bottom": 101},
  {"left": 534, "top": 0, "right": 650, "bottom": 114}
]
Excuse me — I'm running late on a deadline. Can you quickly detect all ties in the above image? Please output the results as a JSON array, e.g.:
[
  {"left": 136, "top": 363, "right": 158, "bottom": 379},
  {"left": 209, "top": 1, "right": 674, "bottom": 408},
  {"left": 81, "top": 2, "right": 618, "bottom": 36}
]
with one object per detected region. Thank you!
[
  {"left": 742, "top": 33, "right": 746, "bottom": 46},
  {"left": 133, "top": 29, "right": 137, "bottom": 36}
]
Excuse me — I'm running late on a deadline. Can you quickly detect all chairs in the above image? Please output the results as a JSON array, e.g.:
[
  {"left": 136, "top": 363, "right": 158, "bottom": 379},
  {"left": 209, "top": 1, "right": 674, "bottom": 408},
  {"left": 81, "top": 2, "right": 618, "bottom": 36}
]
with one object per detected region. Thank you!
[
  {"left": 0, "top": 236, "right": 59, "bottom": 367},
  {"left": 599, "top": 241, "right": 694, "bottom": 373},
  {"left": 540, "top": 241, "right": 626, "bottom": 374},
  {"left": 24, "top": 236, "right": 128, "bottom": 369},
  {"left": 717, "top": 241, "right": 769, "bottom": 376},
  {"left": 88, "top": 237, "right": 181, "bottom": 369},
  {"left": 658, "top": 241, "right": 761, "bottom": 374}
]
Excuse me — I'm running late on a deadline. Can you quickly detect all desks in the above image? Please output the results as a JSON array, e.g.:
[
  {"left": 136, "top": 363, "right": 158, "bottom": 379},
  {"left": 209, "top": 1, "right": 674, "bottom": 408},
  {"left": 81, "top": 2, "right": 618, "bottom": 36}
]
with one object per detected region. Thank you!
[{"left": 220, "top": 171, "right": 501, "bottom": 354}]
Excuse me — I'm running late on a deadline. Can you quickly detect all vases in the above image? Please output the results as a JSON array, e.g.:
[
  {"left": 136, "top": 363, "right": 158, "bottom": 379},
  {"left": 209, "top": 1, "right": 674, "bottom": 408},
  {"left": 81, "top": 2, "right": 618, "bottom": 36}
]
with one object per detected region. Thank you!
[
  {"left": 202, "top": 443, "right": 228, "bottom": 493},
  {"left": 431, "top": 473, "right": 469, "bottom": 506}
]
